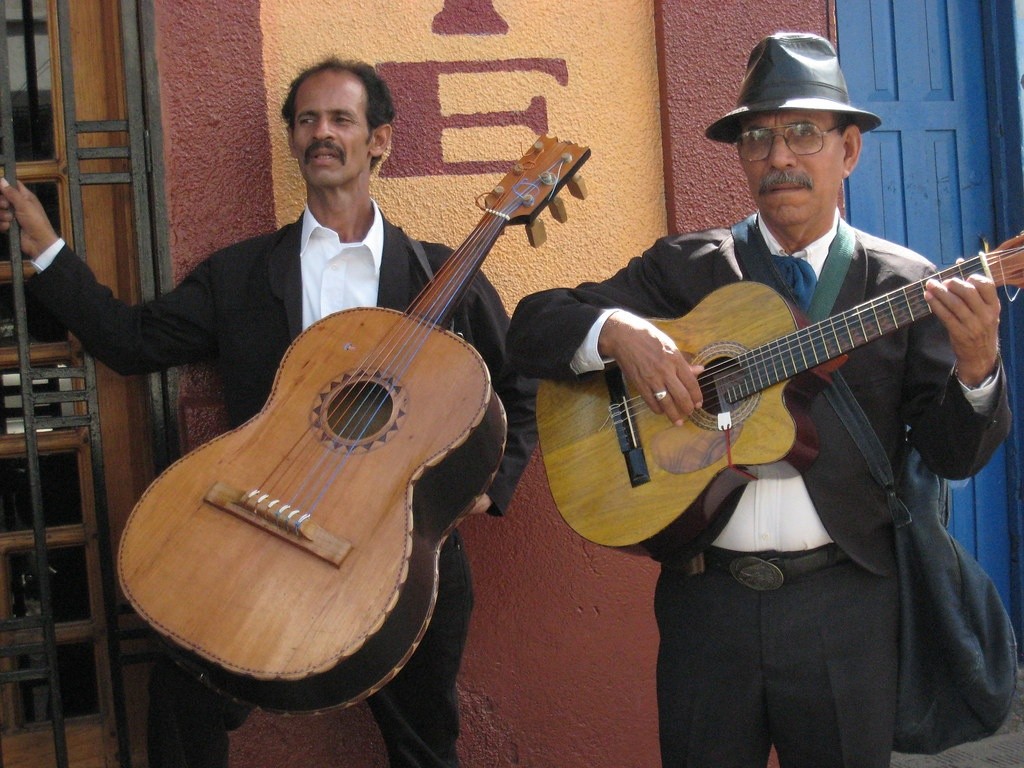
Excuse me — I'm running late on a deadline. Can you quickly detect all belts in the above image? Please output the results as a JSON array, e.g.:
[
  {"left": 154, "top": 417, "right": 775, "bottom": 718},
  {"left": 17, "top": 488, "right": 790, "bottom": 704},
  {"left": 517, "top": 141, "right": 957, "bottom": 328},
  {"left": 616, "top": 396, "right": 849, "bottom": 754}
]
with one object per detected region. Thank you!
[{"left": 704, "top": 545, "right": 846, "bottom": 592}]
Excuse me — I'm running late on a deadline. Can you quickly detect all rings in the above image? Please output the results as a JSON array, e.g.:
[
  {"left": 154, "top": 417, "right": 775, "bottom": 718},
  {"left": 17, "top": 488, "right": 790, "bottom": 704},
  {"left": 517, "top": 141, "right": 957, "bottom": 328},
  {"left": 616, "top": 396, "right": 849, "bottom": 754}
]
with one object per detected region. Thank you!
[{"left": 653, "top": 390, "right": 668, "bottom": 402}]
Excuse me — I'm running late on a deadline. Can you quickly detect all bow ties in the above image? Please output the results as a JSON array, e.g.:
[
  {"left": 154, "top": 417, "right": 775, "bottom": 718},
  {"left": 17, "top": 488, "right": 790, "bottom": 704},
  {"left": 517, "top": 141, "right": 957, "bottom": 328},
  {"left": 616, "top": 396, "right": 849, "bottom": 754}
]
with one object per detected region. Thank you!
[{"left": 772, "top": 254, "right": 817, "bottom": 312}]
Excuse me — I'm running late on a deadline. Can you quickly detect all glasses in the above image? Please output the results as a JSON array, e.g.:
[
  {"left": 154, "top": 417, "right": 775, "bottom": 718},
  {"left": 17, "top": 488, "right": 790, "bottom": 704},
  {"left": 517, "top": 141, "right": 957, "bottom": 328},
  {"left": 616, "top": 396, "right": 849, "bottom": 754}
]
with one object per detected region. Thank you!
[{"left": 736, "top": 120, "right": 849, "bottom": 162}]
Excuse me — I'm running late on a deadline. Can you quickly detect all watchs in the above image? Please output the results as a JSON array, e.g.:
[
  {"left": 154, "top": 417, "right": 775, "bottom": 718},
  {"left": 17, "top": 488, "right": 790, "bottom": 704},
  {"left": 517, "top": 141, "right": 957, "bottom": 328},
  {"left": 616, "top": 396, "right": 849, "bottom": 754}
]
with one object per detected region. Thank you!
[{"left": 954, "top": 355, "right": 999, "bottom": 390}]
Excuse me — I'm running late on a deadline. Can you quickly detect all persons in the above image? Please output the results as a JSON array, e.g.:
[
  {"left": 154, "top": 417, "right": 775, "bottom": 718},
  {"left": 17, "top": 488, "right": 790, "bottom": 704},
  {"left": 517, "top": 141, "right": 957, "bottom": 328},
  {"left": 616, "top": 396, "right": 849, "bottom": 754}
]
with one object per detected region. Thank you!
[
  {"left": 505, "top": 30, "right": 1011, "bottom": 768},
  {"left": 0, "top": 55, "right": 539, "bottom": 768}
]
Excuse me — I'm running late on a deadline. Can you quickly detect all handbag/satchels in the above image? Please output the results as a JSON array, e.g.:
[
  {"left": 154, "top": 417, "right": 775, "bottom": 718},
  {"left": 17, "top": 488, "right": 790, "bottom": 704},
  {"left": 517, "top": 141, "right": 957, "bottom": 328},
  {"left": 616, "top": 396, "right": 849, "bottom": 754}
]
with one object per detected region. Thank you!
[{"left": 890, "top": 442, "right": 1018, "bottom": 754}]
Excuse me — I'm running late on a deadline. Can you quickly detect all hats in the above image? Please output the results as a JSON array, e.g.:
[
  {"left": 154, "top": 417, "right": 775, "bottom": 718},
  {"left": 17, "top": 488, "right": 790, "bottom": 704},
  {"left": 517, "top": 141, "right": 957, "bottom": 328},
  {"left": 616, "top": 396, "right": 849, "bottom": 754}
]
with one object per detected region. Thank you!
[{"left": 705, "top": 32, "right": 882, "bottom": 143}]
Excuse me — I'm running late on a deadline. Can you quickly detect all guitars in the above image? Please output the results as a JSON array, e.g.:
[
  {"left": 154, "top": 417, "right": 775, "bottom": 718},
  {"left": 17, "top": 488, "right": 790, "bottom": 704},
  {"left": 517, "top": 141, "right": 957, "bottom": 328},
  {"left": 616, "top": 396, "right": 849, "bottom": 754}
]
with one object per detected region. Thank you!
[
  {"left": 114, "top": 137, "right": 593, "bottom": 714},
  {"left": 532, "top": 231, "right": 1024, "bottom": 562}
]
